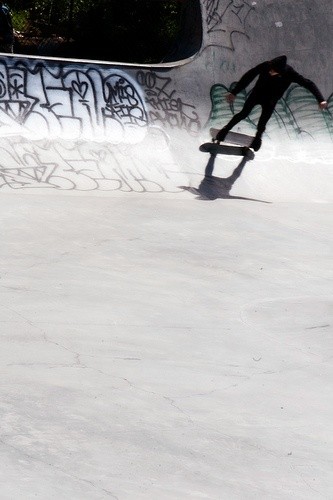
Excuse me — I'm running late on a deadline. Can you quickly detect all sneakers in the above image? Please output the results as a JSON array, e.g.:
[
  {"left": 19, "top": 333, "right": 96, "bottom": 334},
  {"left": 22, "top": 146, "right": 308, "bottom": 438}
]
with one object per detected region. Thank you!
[
  {"left": 244, "top": 144, "right": 259, "bottom": 156},
  {"left": 211, "top": 138, "right": 220, "bottom": 144}
]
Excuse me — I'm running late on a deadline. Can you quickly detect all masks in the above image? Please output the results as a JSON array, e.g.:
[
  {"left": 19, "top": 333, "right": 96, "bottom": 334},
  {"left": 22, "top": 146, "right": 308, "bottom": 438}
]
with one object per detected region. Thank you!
[{"left": 270, "top": 71, "right": 279, "bottom": 77}]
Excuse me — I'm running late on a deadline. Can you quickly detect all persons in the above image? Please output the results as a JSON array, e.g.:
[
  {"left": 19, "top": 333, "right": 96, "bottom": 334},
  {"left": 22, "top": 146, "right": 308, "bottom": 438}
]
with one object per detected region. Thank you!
[{"left": 212, "top": 55, "right": 328, "bottom": 152}]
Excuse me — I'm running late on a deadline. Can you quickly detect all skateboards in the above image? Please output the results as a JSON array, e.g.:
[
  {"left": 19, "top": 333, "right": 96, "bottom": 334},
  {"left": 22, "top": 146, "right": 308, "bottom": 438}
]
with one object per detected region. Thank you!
[{"left": 210, "top": 127, "right": 262, "bottom": 149}]
